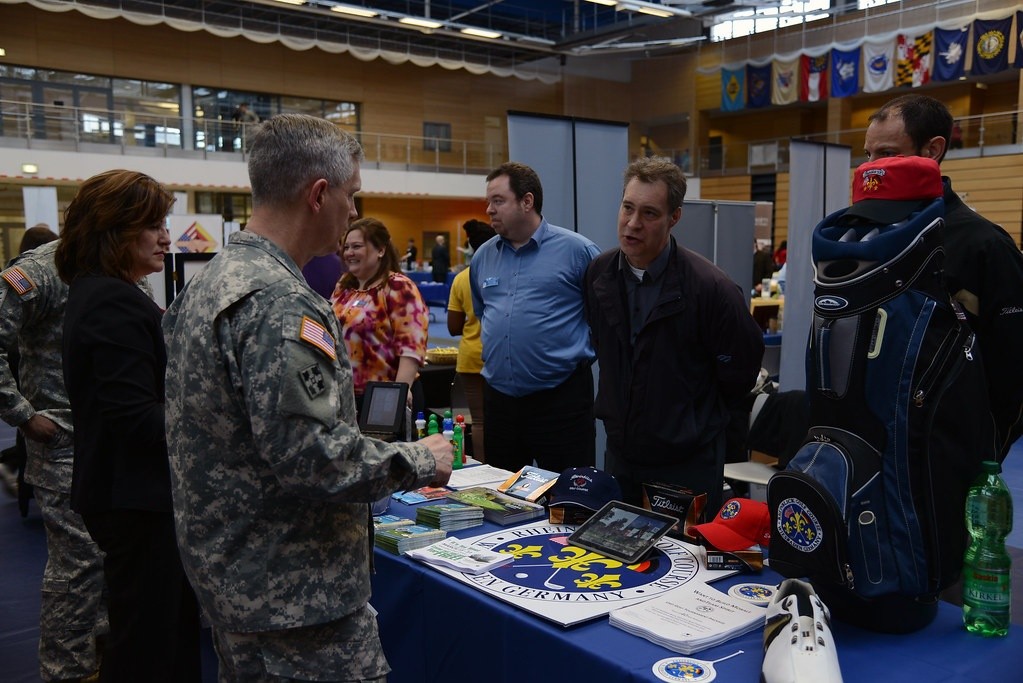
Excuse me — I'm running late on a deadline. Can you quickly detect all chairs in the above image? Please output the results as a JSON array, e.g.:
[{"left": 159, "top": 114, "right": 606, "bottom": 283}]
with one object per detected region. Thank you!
[{"left": 722, "top": 389, "right": 811, "bottom": 485}]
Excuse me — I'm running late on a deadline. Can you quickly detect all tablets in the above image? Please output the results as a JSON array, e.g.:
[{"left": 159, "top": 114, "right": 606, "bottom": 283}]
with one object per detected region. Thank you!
[{"left": 566, "top": 500, "right": 681, "bottom": 564}]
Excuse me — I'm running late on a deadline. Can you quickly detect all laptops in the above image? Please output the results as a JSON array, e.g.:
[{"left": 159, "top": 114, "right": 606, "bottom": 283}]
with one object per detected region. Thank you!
[{"left": 358, "top": 381, "right": 410, "bottom": 442}]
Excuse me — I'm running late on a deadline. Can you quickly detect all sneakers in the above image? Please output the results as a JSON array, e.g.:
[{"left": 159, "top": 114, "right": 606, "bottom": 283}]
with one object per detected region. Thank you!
[{"left": 762, "top": 578, "right": 844, "bottom": 683}]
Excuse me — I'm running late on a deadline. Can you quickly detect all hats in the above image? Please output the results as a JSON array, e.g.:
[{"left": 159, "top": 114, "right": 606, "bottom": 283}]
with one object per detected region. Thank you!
[
  {"left": 836, "top": 153, "right": 946, "bottom": 226},
  {"left": 469, "top": 222, "right": 497, "bottom": 253},
  {"left": 548, "top": 465, "right": 627, "bottom": 512},
  {"left": 695, "top": 497, "right": 771, "bottom": 553}
]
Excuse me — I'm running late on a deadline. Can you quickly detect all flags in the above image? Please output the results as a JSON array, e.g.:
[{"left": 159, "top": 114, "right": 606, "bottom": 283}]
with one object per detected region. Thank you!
[{"left": 720, "top": 10, "right": 1023, "bottom": 112}]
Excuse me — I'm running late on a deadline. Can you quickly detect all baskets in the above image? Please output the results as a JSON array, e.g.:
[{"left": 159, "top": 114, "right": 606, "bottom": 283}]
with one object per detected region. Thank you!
[{"left": 426, "top": 348, "right": 459, "bottom": 366}]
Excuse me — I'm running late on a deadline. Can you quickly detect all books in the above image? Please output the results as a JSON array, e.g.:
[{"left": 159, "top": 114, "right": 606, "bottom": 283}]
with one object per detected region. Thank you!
[{"left": 375, "top": 464, "right": 561, "bottom": 556}]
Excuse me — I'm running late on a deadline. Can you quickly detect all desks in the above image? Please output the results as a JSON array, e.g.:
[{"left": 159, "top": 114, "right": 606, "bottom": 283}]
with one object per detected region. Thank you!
[
  {"left": 420, "top": 363, "right": 462, "bottom": 415},
  {"left": 369, "top": 464, "right": 1023, "bottom": 683},
  {"left": 404, "top": 272, "right": 457, "bottom": 324},
  {"left": 750, "top": 298, "right": 784, "bottom": 319}
]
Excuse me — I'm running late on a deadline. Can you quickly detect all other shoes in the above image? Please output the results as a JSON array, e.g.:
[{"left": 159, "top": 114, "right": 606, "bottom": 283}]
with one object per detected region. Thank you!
[{"left": 0, "top": 461, "right": 19, "bottom": 497}]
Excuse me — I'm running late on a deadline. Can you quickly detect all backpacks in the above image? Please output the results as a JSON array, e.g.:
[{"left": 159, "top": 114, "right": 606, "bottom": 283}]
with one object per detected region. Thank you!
[{"left": 763, "top": 202, "right": 992, "bottom": 638}]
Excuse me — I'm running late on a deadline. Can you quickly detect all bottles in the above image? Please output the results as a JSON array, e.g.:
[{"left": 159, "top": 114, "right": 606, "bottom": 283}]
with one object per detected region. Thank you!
[
  {"left": 414, "top": 411, "right": 465, "bottom": 470},
  {"left": 962, "top": 461, "right": 1017, "bottom": 637}
]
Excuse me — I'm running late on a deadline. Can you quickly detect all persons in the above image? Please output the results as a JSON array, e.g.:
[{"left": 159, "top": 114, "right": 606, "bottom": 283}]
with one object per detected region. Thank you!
[
  {"left": 238, "top": 102, "right": 260, "bottom": 152},
  {"left": 53, "top": 168, "right": 203, "bottom": 683},
  {"left": 0, "top": 208, "right": 153, "bottom": 683},
  {"left": 399, "top": 239, "right": 417, "bottom": 271},
  {"left": 160, "top": 113, "right": 455, "bottom": 683},
  {"left": 446, "top": 218, "right": 499, "bottom": 463},
  {"left": 468, "top": 160, "right": 603, "bottom": 474},
  {"left": 803, "top": 94, "right": 1023, "bottom": 592},
  {"left": 432, "top": 234, "right": 450, "bottom": 283},
  {"left": 753, "top": 237, "right": 787, "bottom": 267},
  {"left": 583, "top": 153, "right": 766, "bottom": 519},
  {"left": 330, "top": 217, "right": 429, "bottom": 426},
  {"left": 20, "top": 226, "right": 59, "bottom": 252}
]
